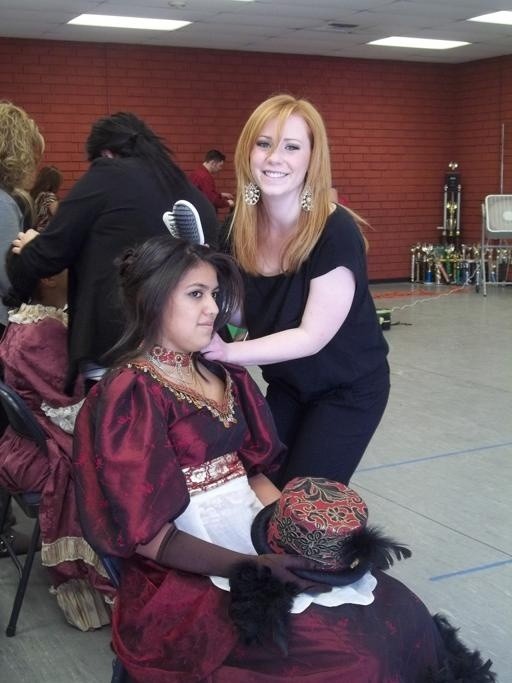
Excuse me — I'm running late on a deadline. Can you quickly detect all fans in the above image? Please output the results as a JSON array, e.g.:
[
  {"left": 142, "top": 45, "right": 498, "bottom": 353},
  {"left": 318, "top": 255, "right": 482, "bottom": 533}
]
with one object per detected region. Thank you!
[{"left": 475, "top": 193, "right": 512, "bottom": 297}]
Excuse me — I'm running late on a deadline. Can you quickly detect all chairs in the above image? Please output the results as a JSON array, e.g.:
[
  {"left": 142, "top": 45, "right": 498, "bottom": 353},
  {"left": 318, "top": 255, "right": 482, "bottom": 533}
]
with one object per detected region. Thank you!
[
  {"left": 1, "top": 384, "right": 74, "bottom": 638},
  {"left": 75, "top": 518, "right": 144, "bottom": 681}
]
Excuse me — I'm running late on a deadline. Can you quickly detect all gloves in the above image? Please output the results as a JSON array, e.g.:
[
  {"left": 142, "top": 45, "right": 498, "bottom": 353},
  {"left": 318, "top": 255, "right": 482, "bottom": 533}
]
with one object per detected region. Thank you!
[{"left": 155, "top": 523, "right": 333, "bottom": 594}]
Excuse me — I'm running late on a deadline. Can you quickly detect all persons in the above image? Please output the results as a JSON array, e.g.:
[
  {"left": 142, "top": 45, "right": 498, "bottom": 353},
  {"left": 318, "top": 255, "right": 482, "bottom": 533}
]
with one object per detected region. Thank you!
[
  {"left": 200, "top": 96, "right": 391, "bottom": 487},
  {"left": 11, "top": 112, "right": 219, "bottom": 400},
  {"left": 74, "top": 235, "right": 495, "bottom": 682},
  {"left": 31, "top": 167, "right": 62, "bottom": 222},
  {"left": 2, "top": 232, "right": 119, "bottom": 630},
  {"left": 0, "top": 97, "right": 45, "bottom": 323},
  {"left": 192, "top": 150, "right": 236, "bottom": 208}
]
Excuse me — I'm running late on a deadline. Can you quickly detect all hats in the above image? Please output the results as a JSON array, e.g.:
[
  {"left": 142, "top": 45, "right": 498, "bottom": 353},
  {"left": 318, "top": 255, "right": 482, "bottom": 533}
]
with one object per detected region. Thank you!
[{"left": 251, "top": 475, "right": 378, "bottom": 586}]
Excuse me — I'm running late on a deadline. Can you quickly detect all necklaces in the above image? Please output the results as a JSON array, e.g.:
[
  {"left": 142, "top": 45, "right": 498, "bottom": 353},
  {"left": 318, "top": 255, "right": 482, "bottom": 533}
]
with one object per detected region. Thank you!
[{"left": 140, "top": 341, "right": 204, "bottom": 398}]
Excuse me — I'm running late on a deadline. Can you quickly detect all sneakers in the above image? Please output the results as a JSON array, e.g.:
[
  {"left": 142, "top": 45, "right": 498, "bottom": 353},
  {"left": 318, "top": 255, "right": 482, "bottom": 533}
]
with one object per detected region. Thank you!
[{"left": 0, "top": 527, "right": 42, "bottom": 557}]
